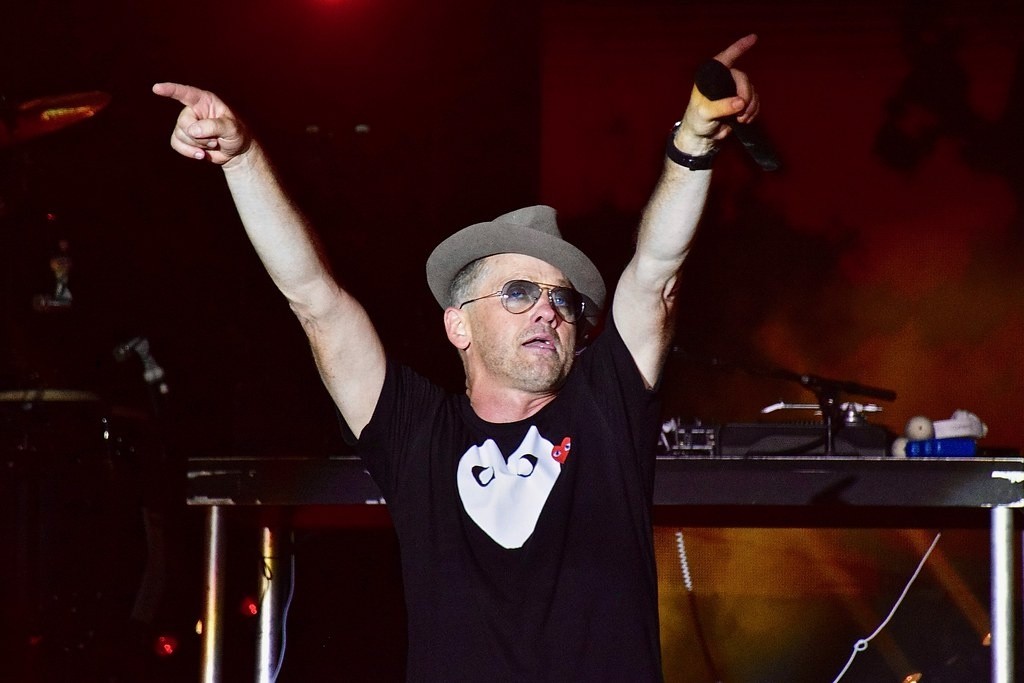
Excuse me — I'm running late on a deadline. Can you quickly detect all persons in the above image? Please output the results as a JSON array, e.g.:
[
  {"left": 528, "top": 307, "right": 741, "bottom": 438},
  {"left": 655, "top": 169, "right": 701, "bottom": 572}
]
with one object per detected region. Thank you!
[{"left": 151, "top": 32, "right": 765, "bottom": 683}]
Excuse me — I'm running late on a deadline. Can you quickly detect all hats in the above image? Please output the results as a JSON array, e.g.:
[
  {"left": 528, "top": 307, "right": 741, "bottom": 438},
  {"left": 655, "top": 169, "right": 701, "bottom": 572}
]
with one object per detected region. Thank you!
[{"left": 426, "top": 203, "right": 607, "bottom": 342}]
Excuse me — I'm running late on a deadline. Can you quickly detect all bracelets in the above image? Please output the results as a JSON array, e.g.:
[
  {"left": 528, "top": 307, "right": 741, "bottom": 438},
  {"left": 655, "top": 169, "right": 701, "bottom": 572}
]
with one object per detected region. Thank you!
[{"left": 665, "top": 120, "right": 721, "bottom": 171}]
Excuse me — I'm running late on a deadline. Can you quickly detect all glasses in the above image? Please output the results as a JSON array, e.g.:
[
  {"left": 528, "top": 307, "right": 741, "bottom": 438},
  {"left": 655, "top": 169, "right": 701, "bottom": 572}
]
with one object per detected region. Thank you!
[{"left": 460, "top": 280, "right": 586, "bottom": 323}]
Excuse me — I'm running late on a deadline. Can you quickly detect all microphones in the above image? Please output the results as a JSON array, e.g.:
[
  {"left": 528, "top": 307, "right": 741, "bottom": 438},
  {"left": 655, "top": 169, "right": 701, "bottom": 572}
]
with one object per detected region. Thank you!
[{"left": 695, "top": 57, "right": 781, "bottom": 173}]
